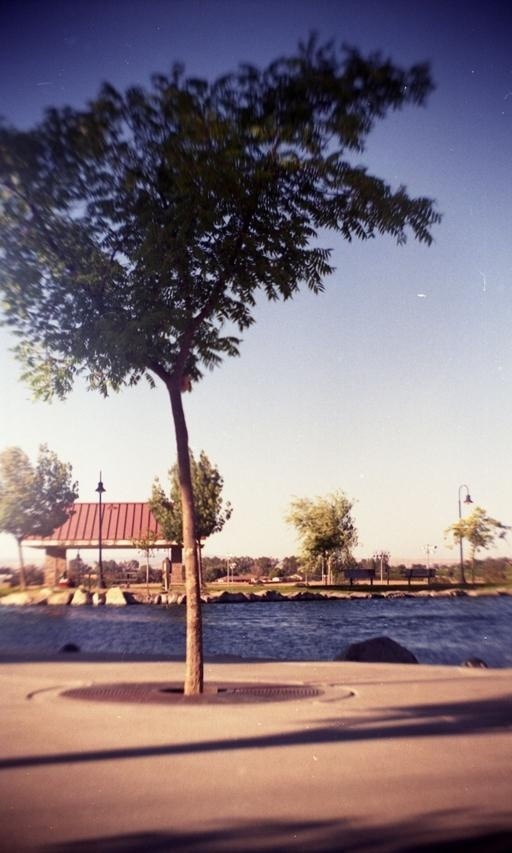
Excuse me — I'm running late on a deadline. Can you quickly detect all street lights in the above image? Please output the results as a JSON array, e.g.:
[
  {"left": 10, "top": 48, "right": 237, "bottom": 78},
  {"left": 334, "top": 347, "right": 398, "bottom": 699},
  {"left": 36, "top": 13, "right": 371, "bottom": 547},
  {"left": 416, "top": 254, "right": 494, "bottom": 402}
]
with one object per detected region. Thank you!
[
  {"left": 458, "top": 483, "right": 474, "bottom": 584},
  {"left": 95, "top": 470, "right": 107, "bottom": 588}
]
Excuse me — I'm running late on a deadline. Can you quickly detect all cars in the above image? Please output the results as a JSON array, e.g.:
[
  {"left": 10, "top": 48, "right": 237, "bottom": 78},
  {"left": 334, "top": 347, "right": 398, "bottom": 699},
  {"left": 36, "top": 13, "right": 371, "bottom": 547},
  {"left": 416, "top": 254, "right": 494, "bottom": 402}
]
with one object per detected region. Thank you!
[{"left": 250, "top": 576, "right": 284, "bottom": 584}]
